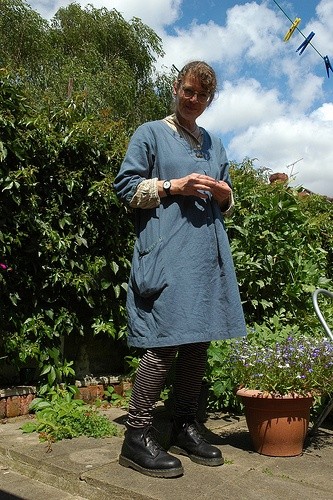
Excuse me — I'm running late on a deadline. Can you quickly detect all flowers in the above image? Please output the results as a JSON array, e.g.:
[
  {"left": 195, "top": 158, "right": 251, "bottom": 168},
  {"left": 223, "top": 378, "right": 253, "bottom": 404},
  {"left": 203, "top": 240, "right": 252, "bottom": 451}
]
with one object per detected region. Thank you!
[{"left": 226, "top": 332, "right": 333, "bottom": 394}]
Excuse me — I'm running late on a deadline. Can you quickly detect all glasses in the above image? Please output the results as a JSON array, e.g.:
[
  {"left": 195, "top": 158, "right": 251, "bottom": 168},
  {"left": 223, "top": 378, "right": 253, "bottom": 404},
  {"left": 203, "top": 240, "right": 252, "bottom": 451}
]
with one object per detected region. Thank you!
[{"left": 180, "top": 83, "right": 211, "bottom": 104}]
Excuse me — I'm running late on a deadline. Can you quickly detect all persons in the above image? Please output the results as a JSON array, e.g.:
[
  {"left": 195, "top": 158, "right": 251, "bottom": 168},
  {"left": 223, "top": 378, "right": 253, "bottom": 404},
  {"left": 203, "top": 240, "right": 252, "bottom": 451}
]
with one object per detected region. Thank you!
[{"left": 112, "top": 59, "right": 250, "bottom": 479}]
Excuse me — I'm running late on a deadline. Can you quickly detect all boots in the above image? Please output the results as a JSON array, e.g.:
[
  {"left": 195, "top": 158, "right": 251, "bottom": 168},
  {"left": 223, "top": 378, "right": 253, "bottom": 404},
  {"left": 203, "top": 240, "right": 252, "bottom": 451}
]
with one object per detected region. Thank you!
[
  {"left": 118, "top": 426, "right": 185, "bottom": 478},
  {"left": 166, "top": 417, "right": 226, "bottom": 467}
]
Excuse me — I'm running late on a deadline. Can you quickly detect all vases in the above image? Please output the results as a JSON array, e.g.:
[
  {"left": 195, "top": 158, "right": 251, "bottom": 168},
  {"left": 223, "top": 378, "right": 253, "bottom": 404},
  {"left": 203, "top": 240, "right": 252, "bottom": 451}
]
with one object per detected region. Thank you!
[{"left": 237, "top": 387, "right": 312, "bottom": 456}]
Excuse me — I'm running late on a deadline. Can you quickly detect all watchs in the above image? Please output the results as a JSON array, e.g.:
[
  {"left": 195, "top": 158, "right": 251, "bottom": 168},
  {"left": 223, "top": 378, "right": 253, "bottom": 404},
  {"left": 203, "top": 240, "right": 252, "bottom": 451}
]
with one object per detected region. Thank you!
[{"left": 161, "top": 178, "right": 172, "bottom": 196}]
